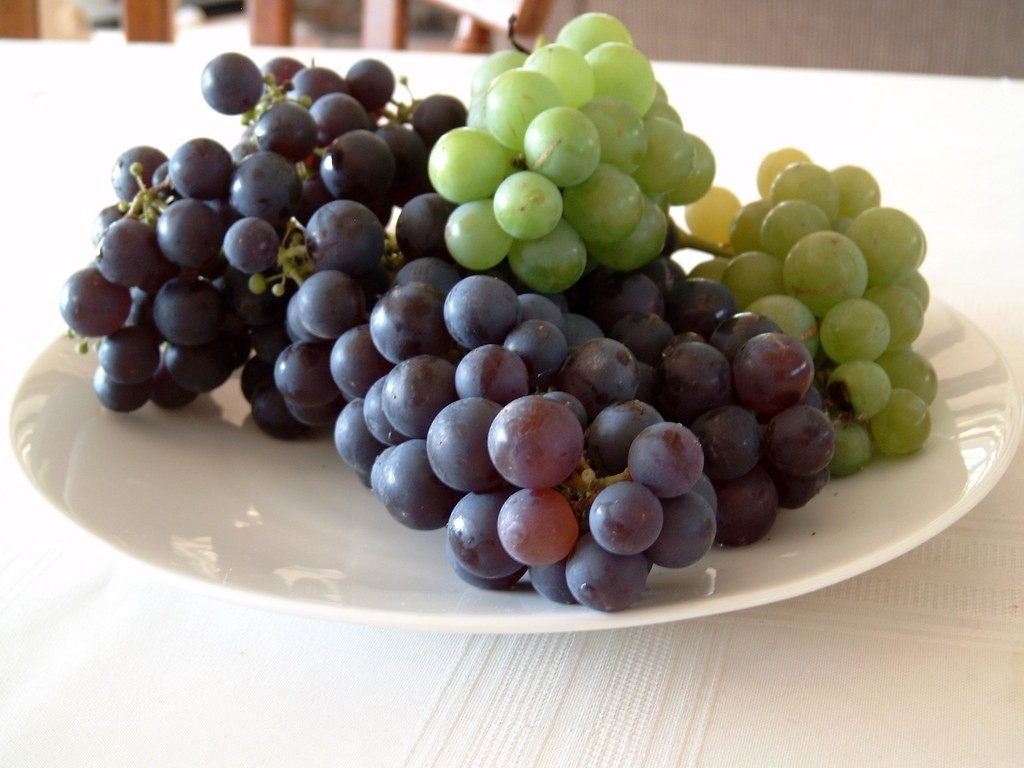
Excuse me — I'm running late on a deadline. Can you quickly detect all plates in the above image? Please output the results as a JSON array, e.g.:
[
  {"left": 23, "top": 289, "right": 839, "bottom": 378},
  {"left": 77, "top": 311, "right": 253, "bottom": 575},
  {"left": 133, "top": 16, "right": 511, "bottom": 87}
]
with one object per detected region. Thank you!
[{"left": 10, "top": 294, "right": 1020, "bottom": 651}]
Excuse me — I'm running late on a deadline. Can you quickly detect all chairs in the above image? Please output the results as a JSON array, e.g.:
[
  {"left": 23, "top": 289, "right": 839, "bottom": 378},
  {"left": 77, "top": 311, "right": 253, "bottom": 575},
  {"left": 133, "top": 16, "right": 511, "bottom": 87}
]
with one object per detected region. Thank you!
[{"left": 0, "top": 0, "right": 554, "bottom": 60}]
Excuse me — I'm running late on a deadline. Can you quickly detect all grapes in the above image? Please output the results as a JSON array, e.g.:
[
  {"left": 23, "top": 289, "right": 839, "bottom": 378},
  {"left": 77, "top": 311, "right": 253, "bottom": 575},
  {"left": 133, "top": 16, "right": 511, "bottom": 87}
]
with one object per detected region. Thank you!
[{"left": 61, "top": 8, "right": 936, "bottom": 611}]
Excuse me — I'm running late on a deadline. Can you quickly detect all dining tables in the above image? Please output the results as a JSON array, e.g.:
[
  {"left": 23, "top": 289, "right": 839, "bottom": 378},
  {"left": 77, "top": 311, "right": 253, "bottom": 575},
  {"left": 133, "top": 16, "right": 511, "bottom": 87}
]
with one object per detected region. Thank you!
[{"left": 0, "top": 34, "right": 1024, "bottom": 768}]
